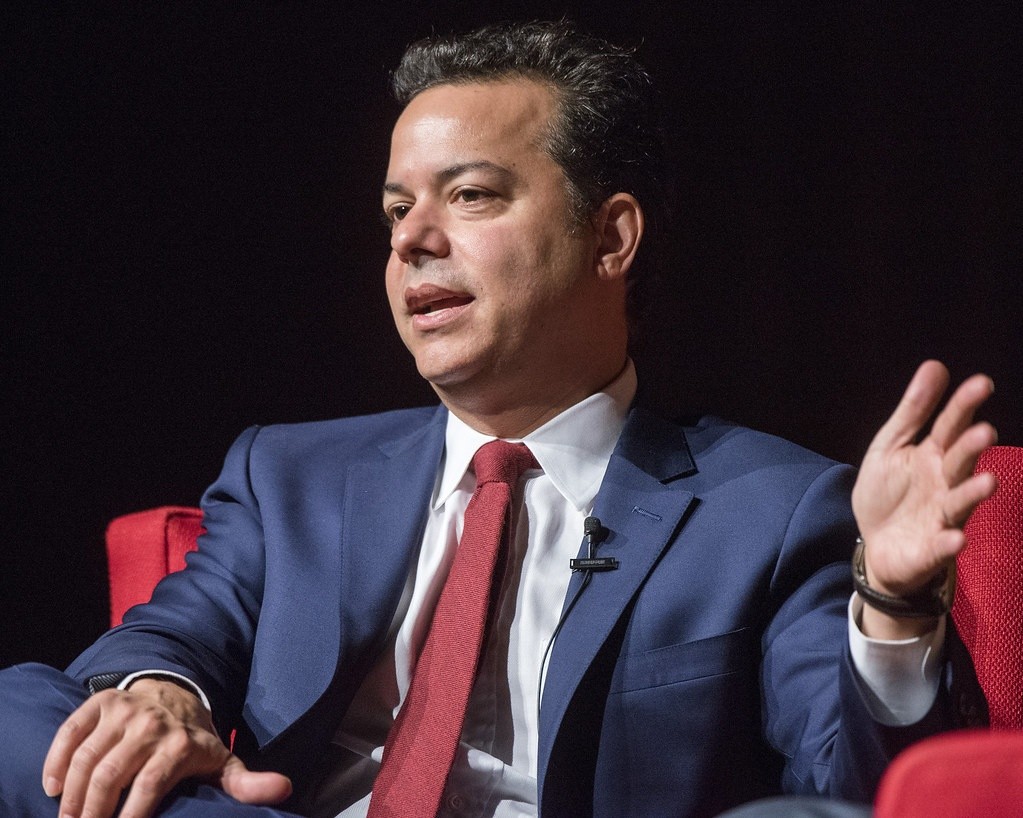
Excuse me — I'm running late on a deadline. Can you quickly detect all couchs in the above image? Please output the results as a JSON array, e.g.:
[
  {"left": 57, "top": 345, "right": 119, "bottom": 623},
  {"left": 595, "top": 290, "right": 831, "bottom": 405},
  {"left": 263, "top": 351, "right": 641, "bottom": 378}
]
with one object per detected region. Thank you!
[{"left": 106, "top": 446, "right": 1022, "bottom": 818}]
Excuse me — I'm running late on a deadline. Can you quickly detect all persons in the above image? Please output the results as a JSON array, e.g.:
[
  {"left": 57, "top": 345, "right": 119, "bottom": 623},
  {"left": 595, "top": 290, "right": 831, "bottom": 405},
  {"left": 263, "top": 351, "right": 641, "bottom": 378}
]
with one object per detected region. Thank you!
[{"left": 0, "top": 21, "right": 1001, "bottom": 816}]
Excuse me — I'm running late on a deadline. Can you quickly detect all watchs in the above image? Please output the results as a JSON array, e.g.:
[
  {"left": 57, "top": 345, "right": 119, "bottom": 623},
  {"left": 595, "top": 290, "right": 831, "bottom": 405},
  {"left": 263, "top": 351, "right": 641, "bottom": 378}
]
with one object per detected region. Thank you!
[{"left": 852, "top": 538, "right": 957, "bottom": 619}]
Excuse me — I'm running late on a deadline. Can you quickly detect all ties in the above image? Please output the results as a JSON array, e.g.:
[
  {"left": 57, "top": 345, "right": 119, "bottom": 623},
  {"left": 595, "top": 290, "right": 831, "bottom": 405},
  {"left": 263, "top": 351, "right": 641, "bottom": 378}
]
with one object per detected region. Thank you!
[{"left": 365, "top": 437, "right": 542, "bottom": 818}]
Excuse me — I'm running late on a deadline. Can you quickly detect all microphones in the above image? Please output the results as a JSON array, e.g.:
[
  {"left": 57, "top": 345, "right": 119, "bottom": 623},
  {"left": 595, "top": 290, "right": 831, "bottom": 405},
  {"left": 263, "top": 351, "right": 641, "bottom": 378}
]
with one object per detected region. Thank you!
[{"left": 584, "top": 517, "right": 601, "bottom": 543}]
